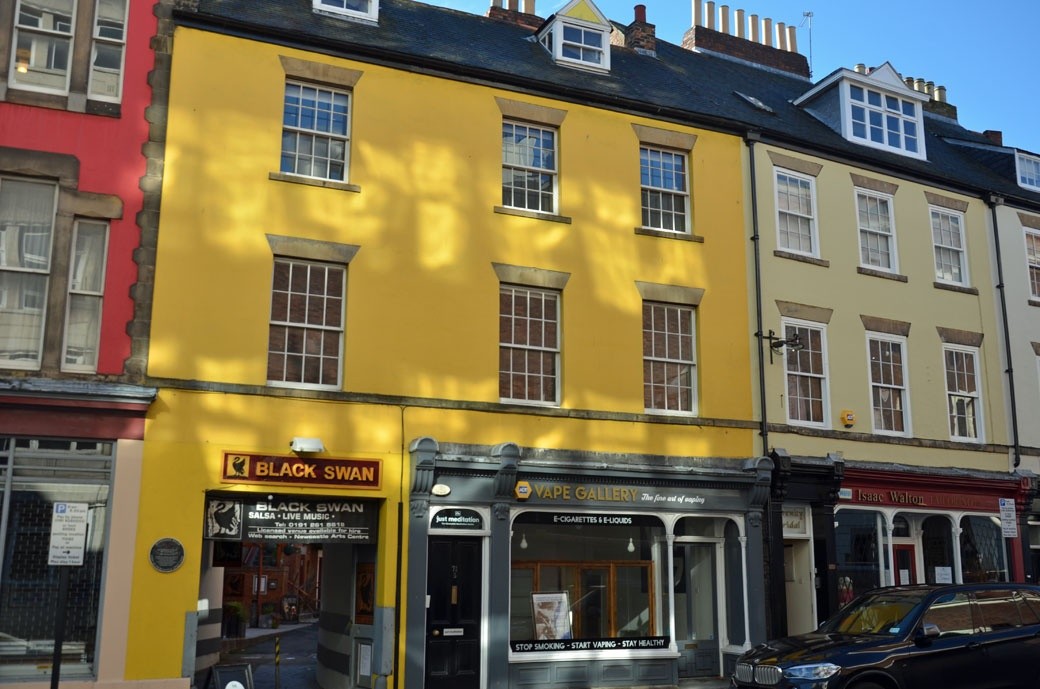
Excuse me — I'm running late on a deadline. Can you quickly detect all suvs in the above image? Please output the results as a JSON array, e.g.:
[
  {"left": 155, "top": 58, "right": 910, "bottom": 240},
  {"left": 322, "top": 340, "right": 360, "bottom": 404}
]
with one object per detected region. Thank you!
[{"left": 730, "top": 584, "right": 1040, "bottom": 689}]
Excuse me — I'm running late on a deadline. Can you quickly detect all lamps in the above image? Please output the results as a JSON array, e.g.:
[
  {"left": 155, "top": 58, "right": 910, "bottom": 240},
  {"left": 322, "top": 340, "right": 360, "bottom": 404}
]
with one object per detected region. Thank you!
[{"left": 289, "top": 438, "right": 325, "bottom": 453}]
